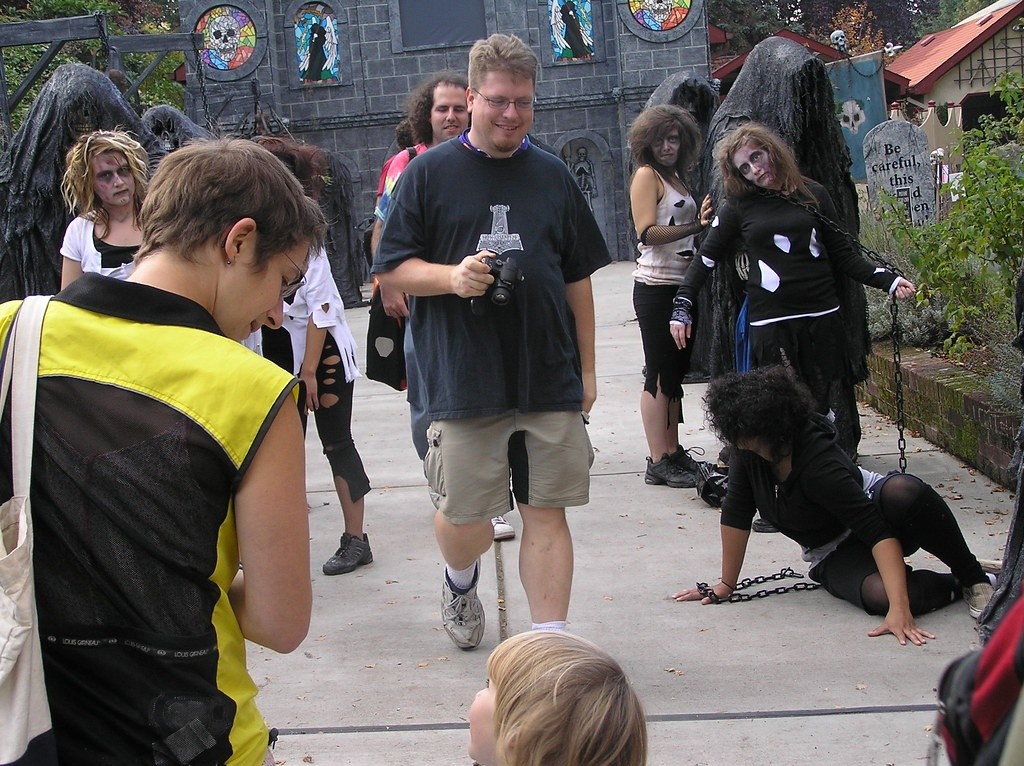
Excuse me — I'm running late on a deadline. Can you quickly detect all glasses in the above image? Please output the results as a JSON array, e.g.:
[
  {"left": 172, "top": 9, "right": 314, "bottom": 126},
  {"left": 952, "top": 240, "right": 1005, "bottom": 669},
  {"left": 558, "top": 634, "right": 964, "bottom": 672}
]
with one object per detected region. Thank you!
[
  {"left": 470, "top": 86, "right": 535, "bottom": 110},
  {"left": 273, "top": 249, "right": 307, "bottom": 300}
]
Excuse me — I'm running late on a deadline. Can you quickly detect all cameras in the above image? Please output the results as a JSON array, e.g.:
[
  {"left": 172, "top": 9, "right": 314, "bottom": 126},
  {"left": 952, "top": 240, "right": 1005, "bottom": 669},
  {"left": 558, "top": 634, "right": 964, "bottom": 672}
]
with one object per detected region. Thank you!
[{"left": 481, "top": 256, "right": 527, "bottom": 305}]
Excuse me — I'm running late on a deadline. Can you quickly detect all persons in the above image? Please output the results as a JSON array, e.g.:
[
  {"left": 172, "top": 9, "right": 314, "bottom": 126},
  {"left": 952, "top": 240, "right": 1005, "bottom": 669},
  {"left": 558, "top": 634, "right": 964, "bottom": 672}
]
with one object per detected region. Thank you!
[
  {"left": 375, "top": 69, "right": 516, "bottom": 540},
  {"left": 672, "top": 364, "right": 1000, "bottom": 646},
  {"left": 60, "top": 130, "right": 151, "bottom": 290},
  {"left": 468, "top": 629, "right": 648, "bottom": 766},
  {"left": 629, "top": 105, "right": 713, "bottom": 488},
  {"left": 252, "top": 136, "right": 372, "bottom": 576},
  {"left": 371, "top": 35, "right": 614, "bottom": 649},
  {"left": 670, "top": 123, "right": 916, "bottom": 533},
  {"left": 0, "top": 138, "right": 326, "bottom": 766}
]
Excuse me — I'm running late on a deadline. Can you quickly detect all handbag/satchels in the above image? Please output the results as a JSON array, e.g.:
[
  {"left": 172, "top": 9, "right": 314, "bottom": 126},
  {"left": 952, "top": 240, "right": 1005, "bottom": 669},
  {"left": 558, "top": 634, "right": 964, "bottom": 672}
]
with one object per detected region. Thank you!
[
  {"left": 0, "top": 293, "right": 52, "bottom": 766},
  {"left": 365, "top": 282, "right": 409, "bottom": 391}
]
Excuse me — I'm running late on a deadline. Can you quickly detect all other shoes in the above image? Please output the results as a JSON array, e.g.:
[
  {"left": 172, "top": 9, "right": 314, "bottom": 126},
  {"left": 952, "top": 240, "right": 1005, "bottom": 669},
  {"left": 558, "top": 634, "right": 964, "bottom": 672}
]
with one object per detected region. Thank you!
[{"left": 961, "top": 583, "right": 997, "bottom": 619}]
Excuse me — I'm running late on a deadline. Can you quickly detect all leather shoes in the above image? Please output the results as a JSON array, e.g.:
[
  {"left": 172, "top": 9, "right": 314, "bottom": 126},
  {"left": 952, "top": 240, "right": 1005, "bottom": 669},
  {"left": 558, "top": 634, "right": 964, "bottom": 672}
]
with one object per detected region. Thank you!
[{"left": 751, "top": 518, "right": 781, "bottom": 533}]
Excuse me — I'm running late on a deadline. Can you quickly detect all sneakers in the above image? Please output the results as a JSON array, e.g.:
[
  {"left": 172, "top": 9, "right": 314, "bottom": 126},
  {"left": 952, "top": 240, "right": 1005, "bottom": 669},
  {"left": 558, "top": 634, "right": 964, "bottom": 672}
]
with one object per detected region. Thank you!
[
  {"left": 440, "top": 556, "right": 486, "bottom": 650},
  {"left": 322, "top": 532, "right": 373, "bottom": 575},
  {"left": 644, "top": 453, "right": 699, "bottom": 487},
  {"left": 673, "top": 444, "right": 705, "bottom": 475},
  {"left": 490, "top": 516, "right": 515, "bottom": 542}
]
